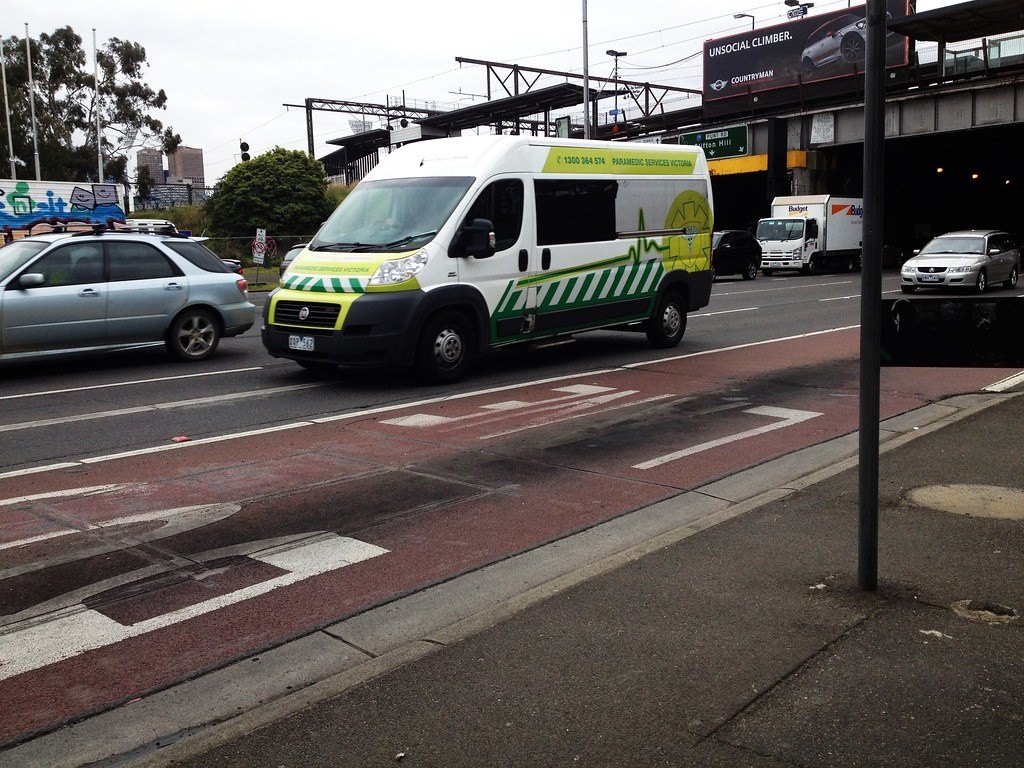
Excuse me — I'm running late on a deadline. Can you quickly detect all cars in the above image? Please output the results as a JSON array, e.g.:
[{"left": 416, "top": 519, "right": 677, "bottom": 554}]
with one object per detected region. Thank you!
[
  {"left": 278, "top": 244, "right": 307, "bottom": 277},
  {"left": 901, "top": 229, "right": 1021, "bottom": 295},
  {"left": 713, "top": 230, "right": 763, "bottom": 280},
  {"left": 802, "top": 11, "right": 894, "bottom": 73},
  {"left": 0, "top": 224, "right": 256, "bottom": 363}
]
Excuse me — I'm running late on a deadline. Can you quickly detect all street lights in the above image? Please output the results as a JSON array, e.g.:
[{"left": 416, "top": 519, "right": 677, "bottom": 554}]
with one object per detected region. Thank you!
[
  {"left": 784, "top": 0, "right": 814, "bottom": 19},
  {"left": 734, "top": 14, "right": 755, "bottom": 30},
  {"left": 606, "top": 50, "right": 628, "bottom": 124}
]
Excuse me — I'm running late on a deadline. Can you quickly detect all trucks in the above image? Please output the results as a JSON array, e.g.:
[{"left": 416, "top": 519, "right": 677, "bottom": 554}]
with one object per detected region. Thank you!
[{"left": 756, "top": 194, "right": 863, "bottom": 276}]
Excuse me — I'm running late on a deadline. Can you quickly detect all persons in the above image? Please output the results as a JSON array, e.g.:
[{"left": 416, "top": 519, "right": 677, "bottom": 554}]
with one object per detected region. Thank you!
[{"left": 382, "top": 198, "right": 432, "bottom": 229}]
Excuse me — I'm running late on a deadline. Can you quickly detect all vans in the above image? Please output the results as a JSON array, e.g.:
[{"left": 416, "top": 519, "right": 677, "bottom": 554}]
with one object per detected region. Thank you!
[{"left": 120, "top": 219, "right": 180, "bottom": 236}]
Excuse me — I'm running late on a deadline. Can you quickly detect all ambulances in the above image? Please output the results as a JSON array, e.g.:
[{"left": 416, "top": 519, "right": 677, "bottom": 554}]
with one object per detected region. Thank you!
[{"left": 261, "top": 134, "right": 709, "bottom": 387}]
